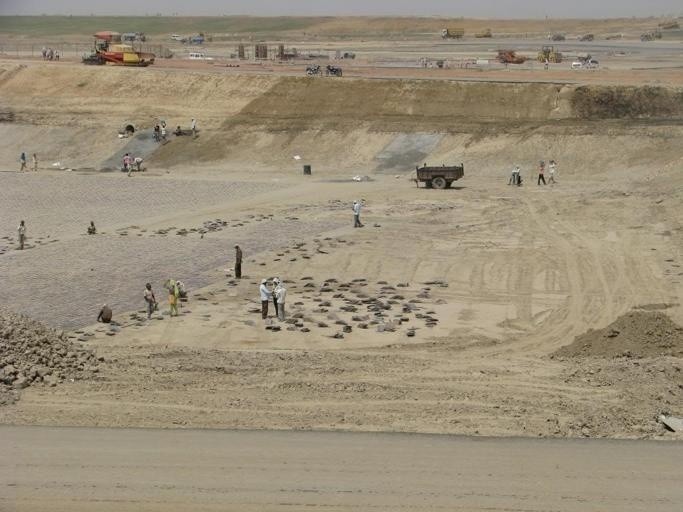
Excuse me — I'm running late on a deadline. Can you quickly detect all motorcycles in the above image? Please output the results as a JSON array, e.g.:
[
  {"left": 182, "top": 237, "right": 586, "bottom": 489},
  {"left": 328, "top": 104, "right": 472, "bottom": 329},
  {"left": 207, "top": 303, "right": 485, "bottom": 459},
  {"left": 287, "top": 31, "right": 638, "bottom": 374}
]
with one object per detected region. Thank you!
[
  {"left": 305, "top": 64, "right": 322, "bottom": 77},
  {"left": 326, "top": 65, "right": 343, "bottom": 76}
]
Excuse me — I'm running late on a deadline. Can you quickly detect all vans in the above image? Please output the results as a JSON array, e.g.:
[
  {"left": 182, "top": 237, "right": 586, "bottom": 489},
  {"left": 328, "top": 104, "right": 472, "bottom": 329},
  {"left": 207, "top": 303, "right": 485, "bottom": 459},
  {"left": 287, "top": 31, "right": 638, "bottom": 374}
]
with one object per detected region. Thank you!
[{"left": 170, "top": 35, "right": 179, "bottom": 40}]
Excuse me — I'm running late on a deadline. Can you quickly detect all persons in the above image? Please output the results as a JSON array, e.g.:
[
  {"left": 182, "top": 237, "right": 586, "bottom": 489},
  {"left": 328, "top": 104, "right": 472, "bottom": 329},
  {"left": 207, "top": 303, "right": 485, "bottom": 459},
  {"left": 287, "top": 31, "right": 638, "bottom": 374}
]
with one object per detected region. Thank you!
[
  {"left": 175, "top": 280, "right": 187, "bottom": 301},
  {"left": 40, "top": 48, "right": 59, "bottom": 62},
  {"left": 86, "top": 221, "right": 95, "bottom": 235},
  {"left": 543, "top": 57, "right": 549, "bottom": 70},
  {"left": 352, "top": 201, "right": 363, "bottom": 228},
  {"left": 153, "top": 118, "right": 199, "bottom": 144},
  {"left": 18, "top": 153, "right": 27, "bottom": 173},
  {"left": 142, "top": 282, "right": 156, "bottom": 318},
  {"left": 274, "top": 280, "right": 286, "bottom": 321},
  {"left": 163, "top": 278, "right": 178, "bottom": 317},
  {"left": 122, "top": 152, "right": 142, "bottom": 176},
  {"left": 29, "top": 153, "right": 37, "bottom": 170},
  {"left": 16, "top": 220, "right": 25, "bottom": 249},
  {"left": 271, "top": 277, "right": 278, "bottom": 316},
  {"left": 507, "top": 159, "right": 557, "bottom": 187},
  {"left": 259, "top": 279, "right": 271, "bottom": 319},
  {"left": 232, "top": 245, "right": 242, "bottom": 279},
  {"left": 96, "top": 303, "right": 112, "bottom": 322}
]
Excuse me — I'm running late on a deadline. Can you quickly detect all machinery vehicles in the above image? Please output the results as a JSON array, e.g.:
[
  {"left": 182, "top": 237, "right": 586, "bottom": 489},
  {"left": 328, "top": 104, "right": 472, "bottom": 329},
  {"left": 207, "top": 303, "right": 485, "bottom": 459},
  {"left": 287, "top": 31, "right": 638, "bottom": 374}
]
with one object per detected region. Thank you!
[
  {"left": 535, "top": 44, "right": 561, "bottom": 64},
  {"left": 81, "top": 31, "right": 155, "bottom": 68},
  {"left": 92, "top": 31, "right": 146, "bottom": 42},
  {"left": 496, "top": 50, "right": 523, "bottom": 64}
]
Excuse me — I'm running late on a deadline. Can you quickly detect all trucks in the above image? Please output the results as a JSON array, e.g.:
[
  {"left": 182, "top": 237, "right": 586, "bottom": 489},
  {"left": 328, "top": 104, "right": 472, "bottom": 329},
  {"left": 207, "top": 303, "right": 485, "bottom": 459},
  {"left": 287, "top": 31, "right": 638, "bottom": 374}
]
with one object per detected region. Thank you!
[{"left": 440, "top": 27, "right": 465, "bottom": 39}]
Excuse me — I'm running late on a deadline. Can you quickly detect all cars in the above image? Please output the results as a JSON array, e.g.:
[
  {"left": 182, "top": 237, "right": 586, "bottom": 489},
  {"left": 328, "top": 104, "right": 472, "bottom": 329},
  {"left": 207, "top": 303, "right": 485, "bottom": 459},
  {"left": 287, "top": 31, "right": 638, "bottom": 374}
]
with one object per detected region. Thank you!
[
  {"left": 570, "top": 61, "right": 582, "bottom": 70},
  {"left": 585, "top": 58, "right": 598, "bottom": 68}
]
